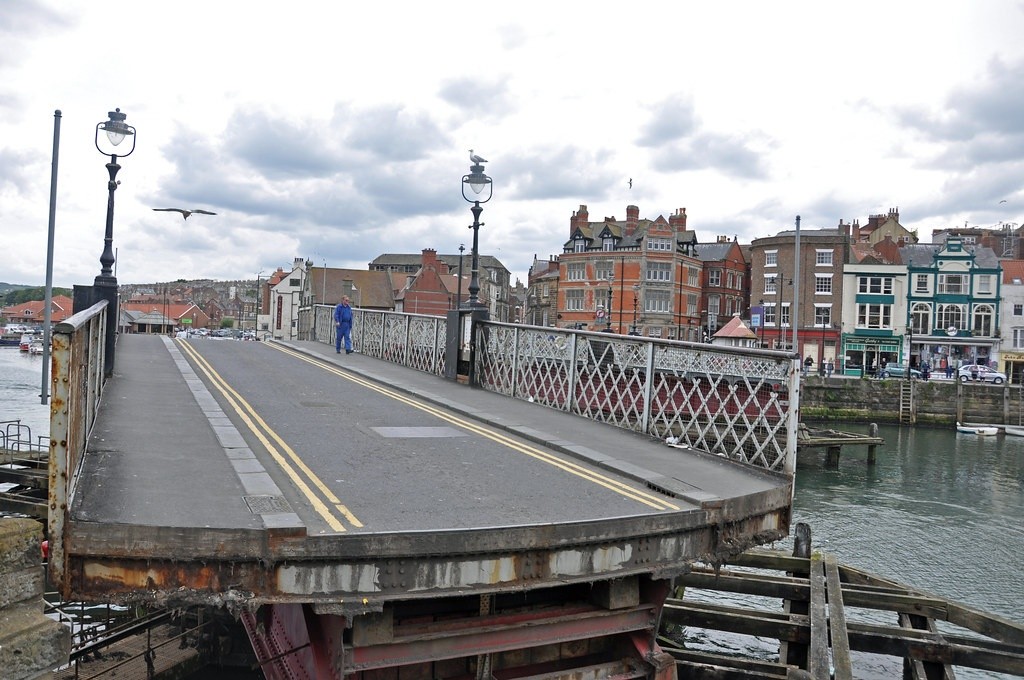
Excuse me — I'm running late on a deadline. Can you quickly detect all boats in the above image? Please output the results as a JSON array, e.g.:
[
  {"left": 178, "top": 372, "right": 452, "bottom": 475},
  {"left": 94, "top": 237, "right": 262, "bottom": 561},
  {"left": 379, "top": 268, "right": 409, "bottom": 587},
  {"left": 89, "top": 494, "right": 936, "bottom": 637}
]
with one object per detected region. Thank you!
[
  {"left": 0, "top": 324, "right": 54, "bottom": 357},
  {"left": 1005, "top": 426, "right": 1024, "bottom": 438},
  {"left": 956, "top": 424, "right": 999, "bottom": 437}
]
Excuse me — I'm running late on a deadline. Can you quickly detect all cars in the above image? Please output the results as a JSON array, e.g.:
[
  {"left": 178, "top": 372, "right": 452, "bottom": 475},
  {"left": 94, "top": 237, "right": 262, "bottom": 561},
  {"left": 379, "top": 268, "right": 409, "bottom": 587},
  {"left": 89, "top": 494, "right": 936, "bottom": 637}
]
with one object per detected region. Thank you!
[
  {"left": 170, "top": 326, "right": 258, "bottom": 341},
  {"left": 958, "top": 364, "right": 1007, "bottom": 385},
  {"left": 881, "top": 362, "right": 923, "bottom": 380}
]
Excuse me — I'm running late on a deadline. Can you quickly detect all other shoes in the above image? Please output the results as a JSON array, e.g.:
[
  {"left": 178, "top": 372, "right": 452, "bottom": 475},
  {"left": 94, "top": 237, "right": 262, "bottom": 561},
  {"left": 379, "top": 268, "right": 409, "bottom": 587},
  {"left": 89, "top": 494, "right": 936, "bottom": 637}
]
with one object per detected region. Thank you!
[
  {"left": 346, "top": 350, "right": 353, "bottom": 354},
  {"left": 337, "top": 351, "right": 341, "bottom": 353}
]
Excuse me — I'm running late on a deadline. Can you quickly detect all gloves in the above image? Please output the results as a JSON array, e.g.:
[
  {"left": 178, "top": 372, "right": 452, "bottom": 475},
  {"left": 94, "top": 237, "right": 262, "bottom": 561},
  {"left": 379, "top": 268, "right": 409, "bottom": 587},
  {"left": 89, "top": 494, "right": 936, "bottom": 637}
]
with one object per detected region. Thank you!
[{"left": 336, "top": 322, "right": 340, "bottom": 327}]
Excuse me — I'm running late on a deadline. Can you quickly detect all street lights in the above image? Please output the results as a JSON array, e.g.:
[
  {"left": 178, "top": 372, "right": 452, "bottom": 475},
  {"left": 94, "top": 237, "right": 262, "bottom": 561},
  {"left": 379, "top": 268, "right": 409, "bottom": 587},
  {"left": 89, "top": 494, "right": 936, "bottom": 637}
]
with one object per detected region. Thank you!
[
  {"left": 768, "top": 272, "right": 793, "bottom": 350},
  {"left": 255, "top": 270, "right": 265, "bottom": 337},
  {"left": 461, "top": 164, "right": 493, "bottom": 378},
  {"left": 757, "top": 299, "right": 771, "bottom": 348},
  {"left": 457, "top": 246, "right": 466, "bottom": 309},
  {"left": 600, "top": 271, "right": 615, "bottom": 333},
  {"left": 627, "top": 282, "right": 643, "bottom": 336},
  {"left": 93, "top": 107, "right": 136, "bottom": 379}
]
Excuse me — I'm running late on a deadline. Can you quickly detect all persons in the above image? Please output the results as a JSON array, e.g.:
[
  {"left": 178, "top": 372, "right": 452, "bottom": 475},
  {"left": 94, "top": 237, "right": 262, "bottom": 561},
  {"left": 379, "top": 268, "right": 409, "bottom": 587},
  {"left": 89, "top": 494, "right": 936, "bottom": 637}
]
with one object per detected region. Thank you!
[
  {"left": 880, "top": 358, "right": 887, "bottom": 379},
  {"left": 823, "top": 357, "right": 833, "bottom": 377},
  {"left": 870, "top": 358, "right": 877, "bottom": 378},
  {"left": 972, "top": 366, "right": 986, "bottom": 386},
  {"left": 334, "top": 295, "right": 354, "bottom": 354},
  {"left": 922, "top": 361, "right": 931, "bottom": 382},
  {"left": 806, "top": 354, "right": 814, "bottom": 372}
]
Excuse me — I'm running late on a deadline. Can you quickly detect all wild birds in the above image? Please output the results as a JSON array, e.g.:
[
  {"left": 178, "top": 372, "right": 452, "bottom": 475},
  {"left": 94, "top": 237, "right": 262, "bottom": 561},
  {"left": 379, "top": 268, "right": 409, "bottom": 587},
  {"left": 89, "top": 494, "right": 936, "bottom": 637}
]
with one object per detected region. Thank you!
[
  {"left": 469, "top": 149, "right": 488, "bottom": 166},
  {"left": 152, "top": 208, "right": 216, "bottom": 220},
  {"left": 627, "top": 178, "right": 632, "bottom": 188}
]
